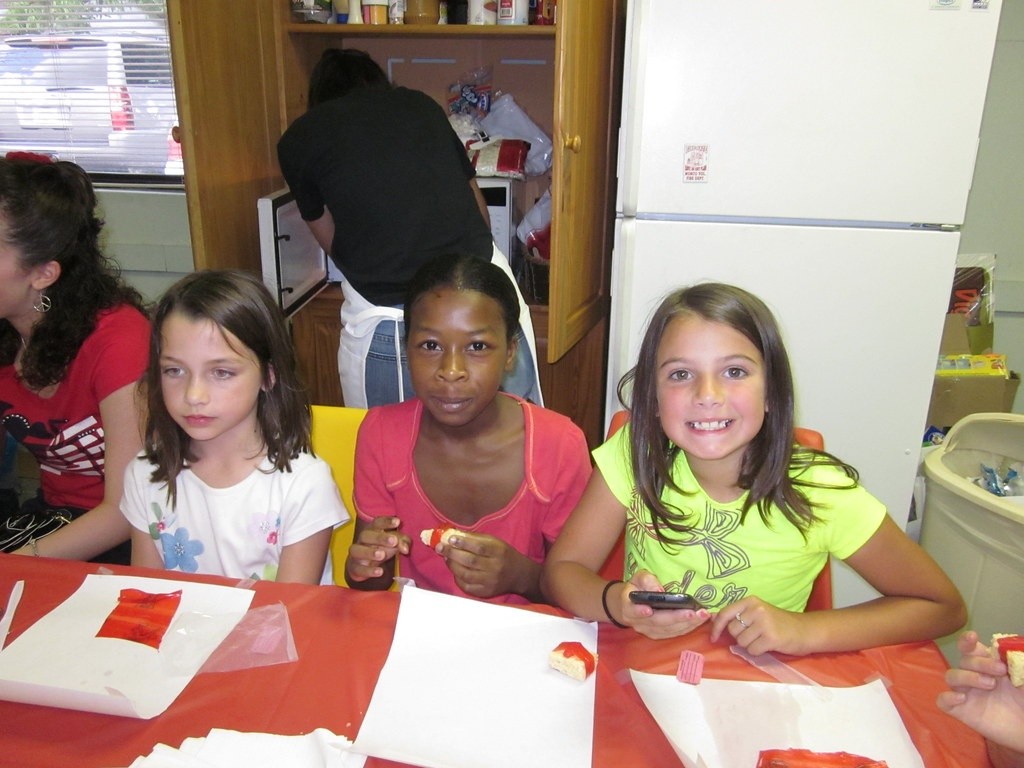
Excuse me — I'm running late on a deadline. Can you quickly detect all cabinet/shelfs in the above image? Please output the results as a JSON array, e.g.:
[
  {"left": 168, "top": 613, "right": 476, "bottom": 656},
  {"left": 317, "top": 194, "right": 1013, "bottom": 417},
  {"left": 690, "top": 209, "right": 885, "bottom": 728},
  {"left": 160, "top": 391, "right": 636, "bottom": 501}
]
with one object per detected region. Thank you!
[{"left": 164, "top": 0, "right": 625, "bottom": 473}]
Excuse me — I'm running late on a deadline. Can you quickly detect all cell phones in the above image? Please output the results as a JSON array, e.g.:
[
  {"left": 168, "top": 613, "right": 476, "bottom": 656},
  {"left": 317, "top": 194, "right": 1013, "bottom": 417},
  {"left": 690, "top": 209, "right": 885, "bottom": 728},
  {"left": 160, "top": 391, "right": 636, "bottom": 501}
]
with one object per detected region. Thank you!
[{"left": 627, "top": 588, "right": 706, "bottom": 613}]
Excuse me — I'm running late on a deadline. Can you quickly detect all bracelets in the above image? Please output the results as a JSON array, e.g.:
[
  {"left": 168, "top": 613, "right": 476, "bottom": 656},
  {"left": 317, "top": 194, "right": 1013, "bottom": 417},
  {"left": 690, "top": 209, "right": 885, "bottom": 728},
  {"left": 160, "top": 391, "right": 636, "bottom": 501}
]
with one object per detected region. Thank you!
[
  {"left": 600, "top": 580, "right": 628, "bottom": 628},
  {"left": 30, "top": 538, "right": 40, "bottom": 558}
]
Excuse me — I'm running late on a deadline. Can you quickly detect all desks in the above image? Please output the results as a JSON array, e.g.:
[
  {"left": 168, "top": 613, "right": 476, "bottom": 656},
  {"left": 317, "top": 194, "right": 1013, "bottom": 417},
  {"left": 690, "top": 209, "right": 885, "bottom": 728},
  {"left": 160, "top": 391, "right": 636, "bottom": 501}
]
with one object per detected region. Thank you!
[{"left": 0, "top": 550, "right": 990, "bottom": 768}]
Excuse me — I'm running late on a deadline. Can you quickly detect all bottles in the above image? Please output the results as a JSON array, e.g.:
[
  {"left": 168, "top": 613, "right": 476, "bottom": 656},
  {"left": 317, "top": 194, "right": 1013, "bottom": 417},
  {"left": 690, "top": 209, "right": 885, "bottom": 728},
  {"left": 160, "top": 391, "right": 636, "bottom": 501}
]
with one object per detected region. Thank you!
[{"left": 329, "top": 0, "right": 557, "bottom": 27}]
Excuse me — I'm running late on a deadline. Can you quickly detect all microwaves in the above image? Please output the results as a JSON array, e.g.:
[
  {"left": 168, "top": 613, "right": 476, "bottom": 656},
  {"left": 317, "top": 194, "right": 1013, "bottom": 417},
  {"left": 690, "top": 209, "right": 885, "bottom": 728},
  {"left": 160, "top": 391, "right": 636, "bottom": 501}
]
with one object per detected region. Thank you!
[{"left": 256, "top": 174, "right": 527, "bottom": 324}]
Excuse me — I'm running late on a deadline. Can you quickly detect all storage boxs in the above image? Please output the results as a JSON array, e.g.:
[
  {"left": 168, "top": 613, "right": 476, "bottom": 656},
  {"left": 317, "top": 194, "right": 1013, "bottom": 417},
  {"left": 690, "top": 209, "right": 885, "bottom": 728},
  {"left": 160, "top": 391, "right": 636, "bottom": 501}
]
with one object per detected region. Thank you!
[
  {"left": 521, "top": 241, "right": 550, "bottom": 306},
  {"left": 921, "top": 322, "right": 1020, "bottom": 435}
]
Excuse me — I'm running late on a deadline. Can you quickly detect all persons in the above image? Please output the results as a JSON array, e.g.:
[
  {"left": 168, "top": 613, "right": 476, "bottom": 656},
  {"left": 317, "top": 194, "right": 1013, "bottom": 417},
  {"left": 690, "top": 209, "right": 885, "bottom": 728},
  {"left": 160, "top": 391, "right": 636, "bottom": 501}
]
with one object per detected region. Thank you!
[
  {"left": 936, "top": 631, "right": 1024, "bottom": 768},
  {"left": 342, "top": 253, "right": 596, "bottom": 609},
  {"left": 0, "top": 155, "right": 156, "bottom": 568},
  {"left": 270, "top": 49, "right": 545, "bottom": 410},
  {"left": 120, "top": 268, "right": 352, "bottom": 587},
  {"left": 536, "top": 282, "right": 969, "bottom": 656}
]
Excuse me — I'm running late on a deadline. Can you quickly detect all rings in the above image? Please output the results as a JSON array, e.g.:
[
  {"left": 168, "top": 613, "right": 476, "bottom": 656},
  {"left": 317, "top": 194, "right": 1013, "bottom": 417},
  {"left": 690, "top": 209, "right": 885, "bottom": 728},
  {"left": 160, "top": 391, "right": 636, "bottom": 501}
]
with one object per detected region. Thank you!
[{"left": 736, "top": 613, "right": 747, "bottom": 627}]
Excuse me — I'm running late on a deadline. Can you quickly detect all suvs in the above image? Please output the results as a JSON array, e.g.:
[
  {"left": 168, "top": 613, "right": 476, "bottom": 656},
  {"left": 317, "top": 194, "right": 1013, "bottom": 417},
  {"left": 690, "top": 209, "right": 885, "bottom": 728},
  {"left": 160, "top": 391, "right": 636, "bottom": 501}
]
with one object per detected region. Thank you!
[{"left": 0, "top": 36, "right": 185, "bottom": 175}]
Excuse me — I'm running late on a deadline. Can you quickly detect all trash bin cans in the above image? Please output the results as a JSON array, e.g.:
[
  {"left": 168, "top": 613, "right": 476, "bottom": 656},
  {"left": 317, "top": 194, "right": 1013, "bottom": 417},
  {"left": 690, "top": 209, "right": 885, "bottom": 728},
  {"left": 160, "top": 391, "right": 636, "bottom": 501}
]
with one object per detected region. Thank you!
[{"left": 920, "top": 412, "right": 1024, "bottom": 671}]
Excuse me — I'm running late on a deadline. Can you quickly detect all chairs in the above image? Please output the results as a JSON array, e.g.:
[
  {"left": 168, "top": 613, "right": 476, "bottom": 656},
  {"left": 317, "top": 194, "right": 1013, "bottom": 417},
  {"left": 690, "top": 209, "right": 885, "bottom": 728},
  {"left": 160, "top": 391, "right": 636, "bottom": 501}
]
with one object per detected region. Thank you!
[
  {"left": 305, "top": 405, "right": 403, "bottom": 593},
  {"left": 598, "top": 410, "right": 833, "bottom": 613}
]
